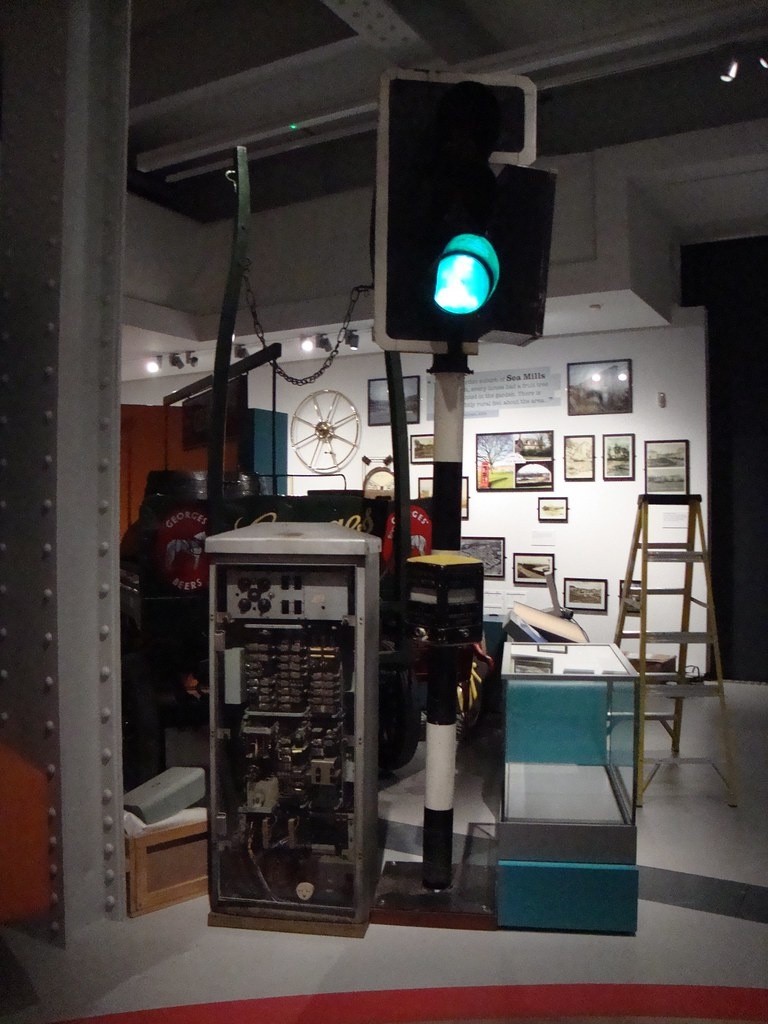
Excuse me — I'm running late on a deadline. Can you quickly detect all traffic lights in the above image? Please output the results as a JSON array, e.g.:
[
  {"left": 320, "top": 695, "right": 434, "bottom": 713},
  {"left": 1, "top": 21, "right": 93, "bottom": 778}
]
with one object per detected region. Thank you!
[{"left": 379, "top": 65, "right": 558, "bottom": 368}]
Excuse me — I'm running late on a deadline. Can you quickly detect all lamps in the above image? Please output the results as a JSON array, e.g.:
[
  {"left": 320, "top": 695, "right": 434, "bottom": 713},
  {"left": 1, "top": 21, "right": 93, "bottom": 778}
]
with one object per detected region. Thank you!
[
  {"left": 185, "top": 351, "right": 198, "bottom": 367},
  {"left": 147, "top": 356, "right": 163, "bottom": 372},
  {"left": 168, "top": 352, "right": 185, "bottom": 370},
  {"left": 346, "top": 330, "right": 359, "bottom": 351},
  {"left": 235, "top": 344, "right": 249, "bottom": 359},
  {"left": 319, "top": 333, "right": 333, "bottom": 353}
]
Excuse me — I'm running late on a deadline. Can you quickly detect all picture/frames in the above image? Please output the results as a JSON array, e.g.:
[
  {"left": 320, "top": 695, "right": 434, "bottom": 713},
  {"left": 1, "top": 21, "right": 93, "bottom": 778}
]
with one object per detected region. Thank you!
[
  {"left": 603, "top": 434, "right": 634, "bottom": 480},
  {"left": 644, "top": 440, "right": 689, "bottom": 496},
  {"left": 564, "top": 435, "right": 595, "bottom": 481},
  {"left": 619, "top": 580, "right": 642, "bottom": 614},
  {"left": 368, "top": 376, "right": 420, "bottom": 426},
  {"left": 475, "top": 430, "right": 554, "bottom": 492},
  {"left": 410, "top": 434, "right": 434, "bottom": 463},
  {"left": 460, "top": 537, "right": 505, "bottom": 578},
  {"left": 537, "top": 496, "right": 568, "bottom": 523},
  {"left": 418, "top": 476, "right": 468, "bottom": 520},
  {"left": 564, "top": 578, "right": 607, "bottom": 611},
  {"left": 513, "top": 553, "right": 555, "bottom": 585},
  {"left": 567, "top": 359, "right": 632, "bottom": 416}
]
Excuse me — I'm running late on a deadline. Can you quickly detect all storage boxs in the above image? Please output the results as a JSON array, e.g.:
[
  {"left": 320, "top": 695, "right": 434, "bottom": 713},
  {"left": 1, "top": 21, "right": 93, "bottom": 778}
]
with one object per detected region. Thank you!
[
  {"left": 498, "top": 640, "right": 642, "bottom": 826},
  {"left": 624, "top": 651, "right": 676, "bottom": 685},
  {"left": 126, "top": 820, "right": 208, "bottom": 918}
]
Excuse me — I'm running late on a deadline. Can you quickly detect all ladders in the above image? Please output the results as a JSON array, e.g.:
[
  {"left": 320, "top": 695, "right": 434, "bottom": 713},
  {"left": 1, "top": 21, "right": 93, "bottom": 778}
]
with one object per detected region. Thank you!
[{"left": 606, "top": 493, "right": 737, "bottom": 806}]
